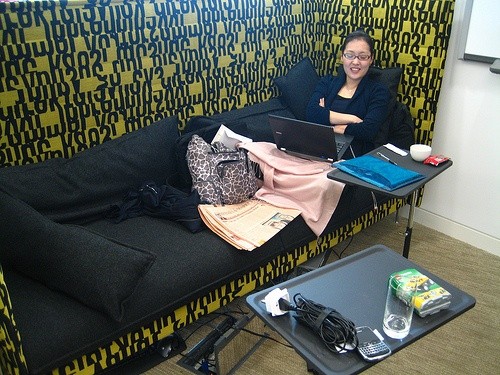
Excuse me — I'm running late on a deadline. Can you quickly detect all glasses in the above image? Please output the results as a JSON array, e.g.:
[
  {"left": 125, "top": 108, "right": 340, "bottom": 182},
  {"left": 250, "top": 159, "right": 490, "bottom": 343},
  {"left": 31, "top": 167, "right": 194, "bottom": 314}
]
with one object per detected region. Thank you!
[{"left": 343, "top": 53, "right": 371, "bottom": 61}]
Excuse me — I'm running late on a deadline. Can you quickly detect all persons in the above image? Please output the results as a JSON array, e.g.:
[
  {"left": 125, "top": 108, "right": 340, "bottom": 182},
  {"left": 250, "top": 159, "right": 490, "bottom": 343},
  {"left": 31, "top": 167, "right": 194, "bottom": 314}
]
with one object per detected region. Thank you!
[{"left": 306, "top": 30, "right": 390, "bottom": 161}]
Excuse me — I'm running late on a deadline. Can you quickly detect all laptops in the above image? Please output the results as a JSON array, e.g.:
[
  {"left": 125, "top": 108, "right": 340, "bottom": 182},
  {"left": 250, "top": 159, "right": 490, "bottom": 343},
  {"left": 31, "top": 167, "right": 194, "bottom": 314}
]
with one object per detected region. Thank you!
[{"left": 268, "top": 113, "right": 355, "bottom": 163}]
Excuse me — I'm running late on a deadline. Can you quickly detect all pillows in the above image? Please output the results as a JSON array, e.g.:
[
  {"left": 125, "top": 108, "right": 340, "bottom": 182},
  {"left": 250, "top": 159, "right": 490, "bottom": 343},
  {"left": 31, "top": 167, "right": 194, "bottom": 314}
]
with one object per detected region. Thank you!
[
  {"left": 273, "top": 57, "right": 320, "bottom": 122},
  {"left": 0, "top": 114, "right": 183, "bottom": 225},
  {"left": 0, "top": 185, "right": 158, "bottom": 325},
  {"left": 337, "top": 64, "right": 402, "bottom": 147},
  {"left": 183, "top": 96, "right": 299, "bottom": 143}
]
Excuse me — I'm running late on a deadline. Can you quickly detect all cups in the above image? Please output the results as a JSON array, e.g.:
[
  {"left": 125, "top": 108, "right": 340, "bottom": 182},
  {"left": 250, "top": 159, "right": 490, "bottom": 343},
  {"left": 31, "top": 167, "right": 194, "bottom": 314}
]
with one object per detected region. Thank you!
[
  {"left": 410, "top": 144, "right": 432, "bottom": 162},
  {"left": 382, "top": 275, "right": 417, "bottom": 339}
]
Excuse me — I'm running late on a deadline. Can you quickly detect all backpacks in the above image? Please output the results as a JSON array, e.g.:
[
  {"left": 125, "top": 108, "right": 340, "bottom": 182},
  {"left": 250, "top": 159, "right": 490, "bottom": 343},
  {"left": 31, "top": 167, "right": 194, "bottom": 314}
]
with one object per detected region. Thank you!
[{"left": 187, "top": 137, "right": 262, "bottom": 204}]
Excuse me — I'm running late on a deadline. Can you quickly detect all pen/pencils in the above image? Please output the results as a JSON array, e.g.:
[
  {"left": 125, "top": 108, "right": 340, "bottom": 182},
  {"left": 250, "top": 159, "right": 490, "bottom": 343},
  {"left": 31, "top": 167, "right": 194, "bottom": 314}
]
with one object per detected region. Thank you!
[{"left": 376, "top": 151, "right": 398, "bottom": 166}]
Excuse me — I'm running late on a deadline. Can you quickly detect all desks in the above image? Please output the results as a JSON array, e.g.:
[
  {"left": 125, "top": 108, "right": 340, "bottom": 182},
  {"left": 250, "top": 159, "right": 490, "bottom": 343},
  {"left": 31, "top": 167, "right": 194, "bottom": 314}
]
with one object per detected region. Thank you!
[
  {"left": 245, "top": 243, "right": 477, "bottom": 375},
  {"left": 326, "top": 144, "right": 454, "bottom": 260}
]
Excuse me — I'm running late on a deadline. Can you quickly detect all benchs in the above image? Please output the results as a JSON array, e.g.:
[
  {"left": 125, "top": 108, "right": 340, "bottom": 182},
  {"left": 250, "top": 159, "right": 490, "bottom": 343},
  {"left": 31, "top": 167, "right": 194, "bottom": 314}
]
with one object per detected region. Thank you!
[{"left": 0, "top": 94, "right": 431, "bottom": 375}]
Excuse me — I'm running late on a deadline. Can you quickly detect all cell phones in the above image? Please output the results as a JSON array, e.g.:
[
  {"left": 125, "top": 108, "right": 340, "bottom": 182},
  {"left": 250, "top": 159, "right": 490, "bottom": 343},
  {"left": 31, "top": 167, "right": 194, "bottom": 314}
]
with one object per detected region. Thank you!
[{"left": 353, "top": 326, "right": 391, "bottom": 363}]
